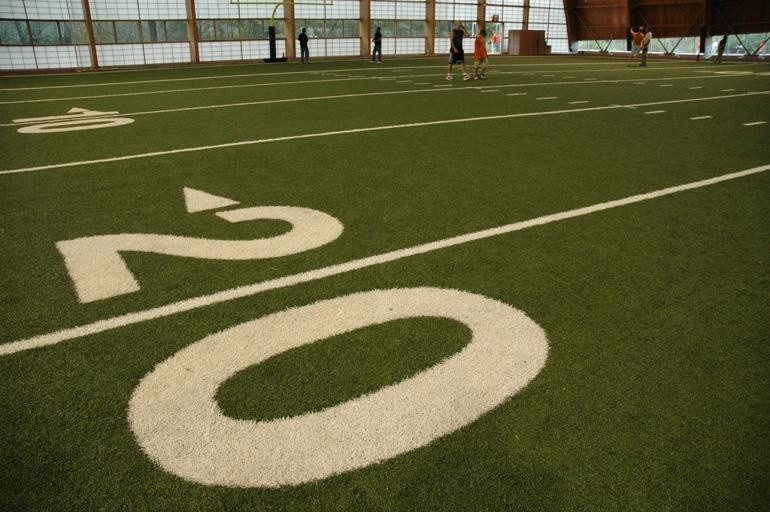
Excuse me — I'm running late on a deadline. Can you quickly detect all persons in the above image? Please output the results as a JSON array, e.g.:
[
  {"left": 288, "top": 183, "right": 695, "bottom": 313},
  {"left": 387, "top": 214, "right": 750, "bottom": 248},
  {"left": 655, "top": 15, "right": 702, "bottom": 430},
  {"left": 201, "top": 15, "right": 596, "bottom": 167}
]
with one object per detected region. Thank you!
[
  {"left": 628, "top": 26, "right": 645, "bottom": 65},
  {"left": 696, "top": 41, "right": 701, "bottom": 53},
  {"left": 492, "top": 31, "right": 498, "bottom": 54},
  {"left": 371, "top": 27, "right": 383, "bottom": 64},
  {"left": 735, "top": 41, "right": 743, "bottom": 54},
  {"left": 641, "top": 26, "right": 653, "bottom": 66},
  {"left": 758, "top": 40, "right": 767, "bottom": 54},
  {"left": 713, "top": 35, "right": 727, "bottom": 64},
  {"left": 298, "top": 28, "right": 311, "bottom": 63},
  {"left": 446, "top": 21, "right": 471, "bottom": 81},
  {"left": 474, "top": 30, "right": 490, "bottom": 80}
]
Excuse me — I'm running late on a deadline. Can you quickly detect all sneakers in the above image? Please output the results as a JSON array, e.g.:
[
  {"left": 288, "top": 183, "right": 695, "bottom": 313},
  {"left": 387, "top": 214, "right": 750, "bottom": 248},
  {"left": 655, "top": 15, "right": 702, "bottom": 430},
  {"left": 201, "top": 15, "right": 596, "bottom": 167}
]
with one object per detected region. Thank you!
[
  {"left": 462, "top": 73, "right": 472, "bottom": 82},
  {"left": 369, "top": 60, "right": 384, "bottom": 64},
  {"left": 445, "top": 75, "right": 455, "bottom": 81},
  {"left": 473, "top": 74, "right": 487, "bottom": 80},
  {"left": 626, "top": 63, "right": 648, "bottom": 67},
  {"left": 301, "top": 59, "right": 310, "bottom": 65}
]
